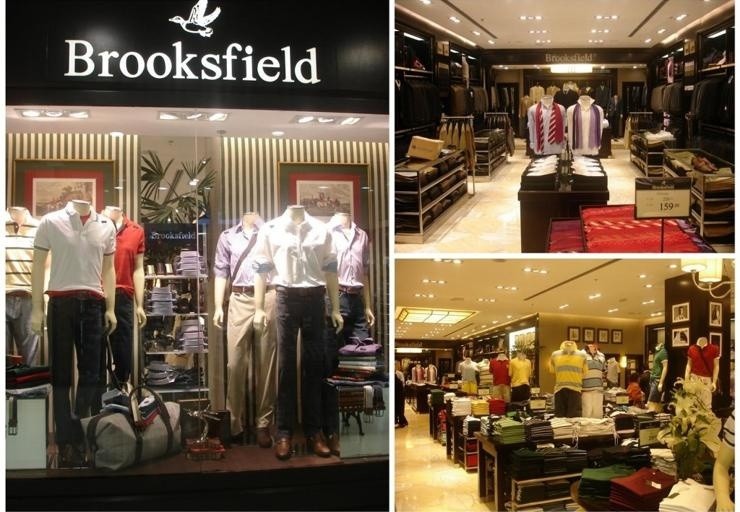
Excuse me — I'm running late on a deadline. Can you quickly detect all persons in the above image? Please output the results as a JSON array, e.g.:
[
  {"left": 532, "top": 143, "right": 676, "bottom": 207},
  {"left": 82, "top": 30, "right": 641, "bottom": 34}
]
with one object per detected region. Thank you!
[
  {"left": 395, "top": 370, "right": 408, "bottom": 428},
  {"left": 6, "top": 206, "right": 52, "bottom": 368},
  {"left": 252, "top": 205, "right": 345, "bottom": 460},
  {"left": 90, "top": 207, "right": 147, "bottom": 390},
  {"left": 30, "top": 200, "right": 117, "bottom": 450},
  {"left": 519, "top": 81, "right": 623, "bottom": 155},
  {"left": 685, "top": 336, "right": 721, "bottom": 409},
  {"left": 712, "top": 410, "right": 735, "bottom": 511},
  {"left": 648, "top": 343, "right": 668, "bottom": 404},
  {"left": 626, "top": 372, "right": 643, "bottom": 402},
  {"left": 711, "top": 304, "right": 720, "bottom": 326},
  {"left": 213, "top": 211, "right": 278, "bottom": 447},
  {"left": 324, "top": 211, "right": 375, "bottom": 456},
  {"left": 412, "top": 340, "right": 622, "bottom": 418},
  {"left": 674, "top": 307, "right": 686, "bottom": 321}
]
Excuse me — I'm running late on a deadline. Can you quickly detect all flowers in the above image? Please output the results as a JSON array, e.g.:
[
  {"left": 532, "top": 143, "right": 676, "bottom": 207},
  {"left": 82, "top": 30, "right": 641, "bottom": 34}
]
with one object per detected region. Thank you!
[{"left": 655, "top": 377, "right": 722, "bottom": 474}]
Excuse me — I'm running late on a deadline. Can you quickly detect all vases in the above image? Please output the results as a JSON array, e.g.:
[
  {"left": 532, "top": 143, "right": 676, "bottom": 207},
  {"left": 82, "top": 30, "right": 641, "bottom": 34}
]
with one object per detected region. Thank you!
[{"left": 672, "top": 444, "right": 697, "bottom": 481}]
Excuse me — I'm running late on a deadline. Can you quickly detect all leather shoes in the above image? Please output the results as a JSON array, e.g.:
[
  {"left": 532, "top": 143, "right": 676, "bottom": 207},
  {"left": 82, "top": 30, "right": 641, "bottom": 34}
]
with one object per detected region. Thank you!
[
  {"left": 305, "top": 432, "right": 331, "bottom": 456},
  {"left": 231, "top": 431, "right": 247, "bottom": 445},
  {"left": 275, "top": 434, "right": 292, "bottom": 459},
  {"left": 256, "top": 429, "right": 273, "bottom": 448},
  {"left": 325, "top": 436, "right": 342, "bottom": 457}
]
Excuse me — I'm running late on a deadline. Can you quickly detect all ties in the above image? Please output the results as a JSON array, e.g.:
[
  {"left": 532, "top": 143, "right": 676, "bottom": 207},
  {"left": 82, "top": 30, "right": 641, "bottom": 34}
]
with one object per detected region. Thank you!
[
  {"left": 571, "top": 101, "right": 601, "bottom": 149},
  {"left": 536, "top": 101, "right": 564, "bottom": 152},
  {"left": 538, "top": 103, "right": 555, "bottom": 155}
]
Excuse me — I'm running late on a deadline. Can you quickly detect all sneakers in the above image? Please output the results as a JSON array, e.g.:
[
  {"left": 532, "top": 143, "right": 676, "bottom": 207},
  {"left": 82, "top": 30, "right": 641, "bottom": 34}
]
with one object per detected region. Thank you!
[{"left": 395, "top": 420, "right": 409, "bottom": 428}]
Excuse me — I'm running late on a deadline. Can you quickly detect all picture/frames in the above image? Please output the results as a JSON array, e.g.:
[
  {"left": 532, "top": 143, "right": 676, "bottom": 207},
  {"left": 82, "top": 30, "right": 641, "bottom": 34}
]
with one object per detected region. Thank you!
[
  {"left": 568, "top": 326, "right": 623, "bottom": 344},
  {"left": 670, "top": 301, "right": 724, "bottom": 356},
  {"left": 11, "top": 159, "right": 118, "bottom": 219},
  {"left": 276, "top": 160, "right": 373, "bottom": 246}
]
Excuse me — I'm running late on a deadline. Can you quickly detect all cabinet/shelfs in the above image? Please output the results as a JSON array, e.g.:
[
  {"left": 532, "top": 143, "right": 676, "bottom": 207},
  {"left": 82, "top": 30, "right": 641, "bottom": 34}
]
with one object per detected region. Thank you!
[
  {"left": 396, "top": 13, "right": 507, "bottom": 243},
  {"left": 629, "top": 14, "right": 735, "bottom": 242},
  {"left": 132, "top": 275, "right": 208, "bottom": 403},
  {"left": 543, "top": 203, "right": 716, "bottom": 253}
]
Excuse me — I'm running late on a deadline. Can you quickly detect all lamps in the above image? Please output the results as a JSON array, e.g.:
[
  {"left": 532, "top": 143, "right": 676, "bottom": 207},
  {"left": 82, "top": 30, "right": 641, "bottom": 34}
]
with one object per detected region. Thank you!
[
  {"left": 681, "top": 257, "right": 735, "bottom": 300},
  {"left": 398, "top": 309, "right": 476, "bottom": 325}
]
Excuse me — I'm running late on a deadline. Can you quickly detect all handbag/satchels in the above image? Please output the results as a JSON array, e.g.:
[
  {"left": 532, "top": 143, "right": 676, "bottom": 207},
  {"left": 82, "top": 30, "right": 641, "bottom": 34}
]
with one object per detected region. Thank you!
[
  {"left": 631, "top": 401, "right": 645, "bottom": 409},
  {"left": 80, "top": 386, "right": 183, "bottom": 472},
  {"left": 7, "top": 365, "right": 54, "bottom": 388},
  {"left": 711, "top": 377, "right": 721, "bottom": 396}
]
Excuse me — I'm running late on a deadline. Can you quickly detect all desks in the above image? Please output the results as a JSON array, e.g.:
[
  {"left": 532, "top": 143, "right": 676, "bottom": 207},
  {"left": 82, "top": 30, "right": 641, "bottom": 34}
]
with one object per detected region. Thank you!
[
  {"left": 518, "top": 154, "right": 608, "bottom": 252},
  {"left": 526, "top": 126, "right": 614, "bottom": 158},
  {"left": 406, "top": 382, "right": 668, "bottom": 512}
]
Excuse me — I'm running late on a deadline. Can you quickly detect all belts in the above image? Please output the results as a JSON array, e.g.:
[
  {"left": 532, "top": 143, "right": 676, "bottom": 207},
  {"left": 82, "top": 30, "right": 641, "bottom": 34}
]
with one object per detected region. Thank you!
[
  {"left": 338, "top": 286, "right": 364, "bottom": 295},
  {"left": 275, "top": 285, "right": 327, "bottom": 297},
  {"left": 232, "top": 286, "right": 269, "bottom": 294},
  {"left": 7, "top": 389, "right": 57, "bottom": 458}
]
